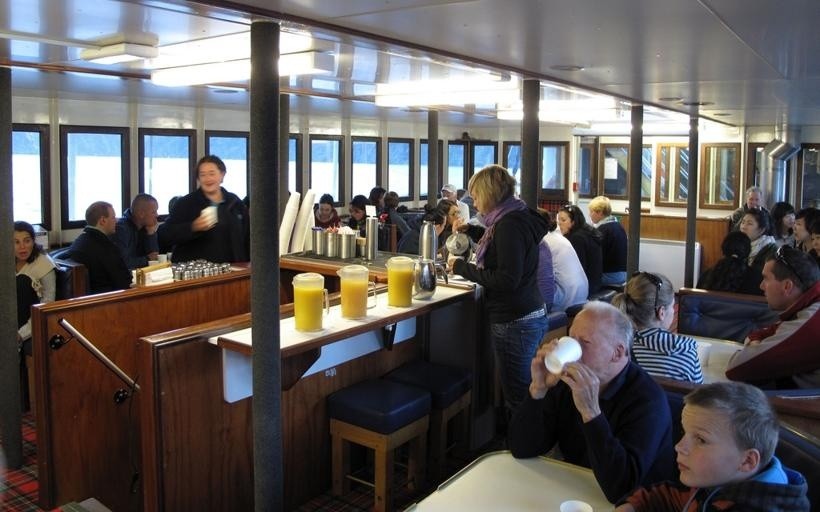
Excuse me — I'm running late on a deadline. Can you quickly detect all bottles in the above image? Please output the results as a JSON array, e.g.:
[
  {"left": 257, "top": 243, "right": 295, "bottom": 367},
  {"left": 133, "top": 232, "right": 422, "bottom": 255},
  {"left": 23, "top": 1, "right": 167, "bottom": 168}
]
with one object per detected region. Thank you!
[{"left": 365, "top": 218, "right": 378, "bottom": 262}]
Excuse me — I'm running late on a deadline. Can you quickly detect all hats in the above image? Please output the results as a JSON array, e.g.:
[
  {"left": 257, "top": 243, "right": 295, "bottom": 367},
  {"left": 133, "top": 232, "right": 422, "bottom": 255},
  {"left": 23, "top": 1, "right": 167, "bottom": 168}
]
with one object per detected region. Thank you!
[{"left": 441, "top": 184, "right": 458, "bottom": 193}]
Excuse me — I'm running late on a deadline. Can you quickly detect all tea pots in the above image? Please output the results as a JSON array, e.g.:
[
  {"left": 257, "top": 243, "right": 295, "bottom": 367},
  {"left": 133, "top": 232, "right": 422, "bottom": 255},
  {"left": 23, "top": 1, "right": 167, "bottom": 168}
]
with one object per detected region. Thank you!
[
  {"left": 418, "top": 213, "right": 438, "bottom": 265},
  {"left": 410, "top": 258, "right": 449, "bottom": 299},
  {"left": 445, "top": 230, "right": 473, "bottom": 265}
]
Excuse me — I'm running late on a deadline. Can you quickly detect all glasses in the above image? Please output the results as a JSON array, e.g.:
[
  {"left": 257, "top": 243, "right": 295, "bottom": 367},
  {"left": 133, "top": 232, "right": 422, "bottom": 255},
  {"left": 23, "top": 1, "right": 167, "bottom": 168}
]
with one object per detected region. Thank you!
[
  {"left": 775, "top": 244, "right": 804, "bottom": 286},
  {"left": 632, "top": 271, "right": 664, "bottom": 319}
]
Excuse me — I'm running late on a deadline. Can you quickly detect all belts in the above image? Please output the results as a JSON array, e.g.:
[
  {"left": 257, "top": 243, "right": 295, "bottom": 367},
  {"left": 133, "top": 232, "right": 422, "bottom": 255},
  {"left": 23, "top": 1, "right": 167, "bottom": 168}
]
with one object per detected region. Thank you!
[{"left": 512, "top": 302, "right": 549, "bottom": 322}]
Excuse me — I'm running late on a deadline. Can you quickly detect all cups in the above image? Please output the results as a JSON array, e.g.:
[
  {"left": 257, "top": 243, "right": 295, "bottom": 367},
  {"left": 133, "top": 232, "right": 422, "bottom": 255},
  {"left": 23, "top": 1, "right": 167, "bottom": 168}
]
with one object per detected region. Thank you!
[
  {"left": 279, "top": 189, "right": 317, "bottom": 255},
  {"left": 157, "top": 254, "right": 168, "bottom": 264},
  {"left": 337, "top": 264, "right": 378, "bottom": 319},
  {"left": 447, "top": 256, "right": 464, "bottom": 264},
  {"left": 291, "top": 273, "right": 330, "bottom": 333},
  {"left": 312, "top": 217, "right": 366, "bottom": 260},
  {"left": 560, "top": 500, "right": 594, "bottom": 512},
  {"left": 543, "top": 335, "right": 583, "bottom": 374},
  {"left": 697, "top": 342, "right": 712, "bottom": 367},
  {"left": 201, "top": 207, "right": 218, "bottom": 224},
  {"left": 149, "top": 260, "right": 159, "bottom": 266},
  {"left": 385, "top": 256, "right": 417, "bottom": 307},
  {"left": 169, "top": 259, "right": 231, "bottom": 281}
]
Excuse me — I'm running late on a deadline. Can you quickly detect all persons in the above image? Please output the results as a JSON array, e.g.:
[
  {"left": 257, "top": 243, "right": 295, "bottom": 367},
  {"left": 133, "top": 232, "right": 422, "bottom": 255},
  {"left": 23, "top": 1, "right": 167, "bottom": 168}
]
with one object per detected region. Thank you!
[
  {"left": 67, "top": 201, "right": 136, "bottom": 291},
  {"left": 535, "top": 196, "right": 629, "bottom": 310},
  {"left": 315, "top": 184, "right": 486, "bottom": 256},
  {"left": 157, "top": 195, "right": 182, "bottom": 253},
  {"left": 506, "top": 301, "right": 678, "bottom": 503},
  {"left": 14, "top": 220, "right": 57, "bottom": 409},
  {"left": 111, "top": 192, "right": 158, "bottom": 269},
  {"left": 611, "top": 380, "right": 813, "bottom": 511},
  {"left": 163, "top": 156, "right": 250, "bottom": 264},
  {"left": 446, "top": 163, "right": 550, "bottom": 432},
  {"left": 611, "top": 183, "right": 819, "bottom": 394}
]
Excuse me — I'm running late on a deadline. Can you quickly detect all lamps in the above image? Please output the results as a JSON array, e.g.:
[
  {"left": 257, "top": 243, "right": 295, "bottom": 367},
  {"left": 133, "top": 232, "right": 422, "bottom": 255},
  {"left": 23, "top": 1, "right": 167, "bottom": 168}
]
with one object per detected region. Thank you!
[
  {"left": 149, "top": 50, "right": 337, "bottom": 89},
  {"left": 79, "top": 40, "right": 157, "bottom": 69}
]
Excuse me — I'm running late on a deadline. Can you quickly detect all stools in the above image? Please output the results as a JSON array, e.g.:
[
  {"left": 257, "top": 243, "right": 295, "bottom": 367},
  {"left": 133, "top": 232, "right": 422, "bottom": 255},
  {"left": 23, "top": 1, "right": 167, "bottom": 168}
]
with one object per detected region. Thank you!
[
  {"left": 324, "top": 374, "right": 432, "bottom": 510},
  {"left": 494, "top": 276, "right": 625, "bottom": 410},
  {"left": 378, "top": 357, "right": 475, "bottom": 476}
]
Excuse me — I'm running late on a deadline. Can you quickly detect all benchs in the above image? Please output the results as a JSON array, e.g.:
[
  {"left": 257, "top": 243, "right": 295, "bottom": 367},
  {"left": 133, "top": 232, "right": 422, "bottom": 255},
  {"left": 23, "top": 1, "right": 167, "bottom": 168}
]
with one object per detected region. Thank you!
[
  {"left": 664, "top": 280, "right": 820, "bottom": 512},
  {"left": 46, "top": 247, "right": 87, "bottom": 301}
]
